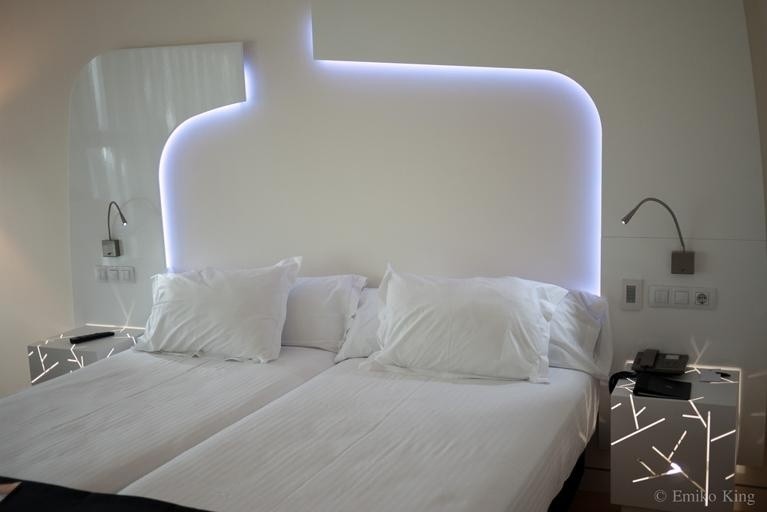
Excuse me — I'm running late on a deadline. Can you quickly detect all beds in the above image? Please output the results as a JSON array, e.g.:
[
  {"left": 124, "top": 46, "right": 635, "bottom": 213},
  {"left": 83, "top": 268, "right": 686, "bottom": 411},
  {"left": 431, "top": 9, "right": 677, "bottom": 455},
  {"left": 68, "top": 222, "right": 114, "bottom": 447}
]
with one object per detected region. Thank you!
[{"left": 1, "top": 350, "right": 608, "bottom": 510}]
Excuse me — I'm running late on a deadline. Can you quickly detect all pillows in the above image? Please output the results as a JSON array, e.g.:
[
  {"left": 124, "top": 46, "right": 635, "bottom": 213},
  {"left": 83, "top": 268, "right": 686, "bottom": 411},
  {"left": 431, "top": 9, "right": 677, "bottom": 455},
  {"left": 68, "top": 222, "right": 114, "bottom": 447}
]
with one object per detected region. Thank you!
[{"left": 133, "top": 254, "right": 605, "bottom": 382}]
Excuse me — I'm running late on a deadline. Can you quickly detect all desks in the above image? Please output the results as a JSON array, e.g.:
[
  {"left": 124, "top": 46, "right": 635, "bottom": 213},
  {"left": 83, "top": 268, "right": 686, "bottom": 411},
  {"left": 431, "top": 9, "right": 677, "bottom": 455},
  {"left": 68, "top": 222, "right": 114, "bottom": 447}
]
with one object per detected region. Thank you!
[
  {"left": 609, "top": 359, "right": 739, "bottom": 511},
  {"left": 28, "top": 325, "right": 146, "bottom": 387}
]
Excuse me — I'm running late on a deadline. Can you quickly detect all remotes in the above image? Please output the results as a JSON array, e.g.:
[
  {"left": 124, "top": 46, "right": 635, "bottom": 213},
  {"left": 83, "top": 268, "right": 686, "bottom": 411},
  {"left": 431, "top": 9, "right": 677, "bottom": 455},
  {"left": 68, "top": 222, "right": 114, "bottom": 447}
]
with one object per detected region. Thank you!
[{"left": 69, "top": 331, "right": 116, "bottom": 343}]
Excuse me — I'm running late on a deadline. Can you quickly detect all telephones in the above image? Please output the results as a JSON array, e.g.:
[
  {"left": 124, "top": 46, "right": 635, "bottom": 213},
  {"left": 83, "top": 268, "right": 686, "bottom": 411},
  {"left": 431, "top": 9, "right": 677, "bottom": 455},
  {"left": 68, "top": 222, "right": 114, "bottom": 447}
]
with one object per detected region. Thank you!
[{"left": 631, "top": 349, "right": 689, "bottom": 378}]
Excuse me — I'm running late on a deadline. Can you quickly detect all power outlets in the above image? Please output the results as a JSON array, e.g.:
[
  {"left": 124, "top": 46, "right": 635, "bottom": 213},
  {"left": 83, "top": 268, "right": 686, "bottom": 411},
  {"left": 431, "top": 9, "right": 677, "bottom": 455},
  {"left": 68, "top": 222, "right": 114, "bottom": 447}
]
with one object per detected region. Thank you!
[{"left": 693, "top": 287, "right": 717, "bottom": 310}]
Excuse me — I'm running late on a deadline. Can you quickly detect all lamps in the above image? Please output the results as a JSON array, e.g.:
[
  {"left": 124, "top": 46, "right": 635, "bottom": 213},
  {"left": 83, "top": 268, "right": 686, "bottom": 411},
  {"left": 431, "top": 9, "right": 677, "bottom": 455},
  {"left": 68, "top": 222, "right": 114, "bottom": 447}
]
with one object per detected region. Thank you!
[
  {"left": 621, "top": 197, "right": 696, "bottom": 275},
  {"left": 102, "top": 200, "right": 131, "bottom": 259}
]
